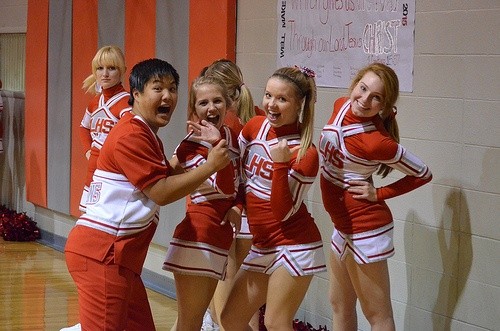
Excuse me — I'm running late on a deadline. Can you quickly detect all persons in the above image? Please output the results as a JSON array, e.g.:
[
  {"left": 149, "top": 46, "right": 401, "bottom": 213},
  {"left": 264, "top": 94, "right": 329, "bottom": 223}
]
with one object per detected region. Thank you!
[
  {"left": 59, "top": 45, "right": 327, "bottom": 331},
  {"left": 319, "top": 63, "right": 434, "bottom": 331}
]
